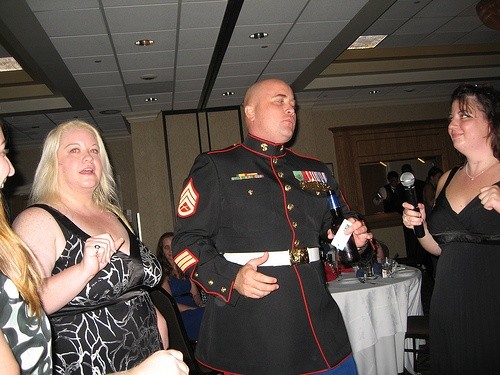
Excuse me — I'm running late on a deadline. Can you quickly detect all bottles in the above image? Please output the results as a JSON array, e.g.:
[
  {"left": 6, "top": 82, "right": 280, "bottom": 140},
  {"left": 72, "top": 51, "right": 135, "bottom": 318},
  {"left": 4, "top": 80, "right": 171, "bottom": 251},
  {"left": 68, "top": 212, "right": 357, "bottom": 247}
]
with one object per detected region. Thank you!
[
  {"left": 320, "top": 240, "right": 337, "bottom": 278},
  {"left": 366, "top": 263, "right": 375, "bottom": 277},
  {"left": 327, "top": 189, "right": 360, "bottom": 266}
]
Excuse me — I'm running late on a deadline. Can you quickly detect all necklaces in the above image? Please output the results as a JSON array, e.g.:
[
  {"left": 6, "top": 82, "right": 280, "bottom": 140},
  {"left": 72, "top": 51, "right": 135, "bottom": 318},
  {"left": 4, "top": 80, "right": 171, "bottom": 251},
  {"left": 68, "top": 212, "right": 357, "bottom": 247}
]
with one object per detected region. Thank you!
[{"left": 466, "top": 162, "right": 498, "bottom": 181}]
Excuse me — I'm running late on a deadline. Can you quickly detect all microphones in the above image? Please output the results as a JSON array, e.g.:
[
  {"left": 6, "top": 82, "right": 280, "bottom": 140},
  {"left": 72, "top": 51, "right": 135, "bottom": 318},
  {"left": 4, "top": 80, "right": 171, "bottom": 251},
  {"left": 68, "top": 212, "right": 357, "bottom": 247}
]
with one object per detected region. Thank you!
[{"left": 399, "top": 172, "right": 426, "bottom": 238}]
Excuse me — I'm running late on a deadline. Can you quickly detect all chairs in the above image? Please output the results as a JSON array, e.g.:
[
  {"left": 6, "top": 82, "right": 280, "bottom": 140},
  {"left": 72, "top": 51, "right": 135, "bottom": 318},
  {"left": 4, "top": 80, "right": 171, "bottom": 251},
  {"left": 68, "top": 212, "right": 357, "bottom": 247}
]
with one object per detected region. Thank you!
[
  {"left": 405, "top": 315, "right": 429, "bottom": 374},
  {"left": 149, "top": 287, "right": 220, "bottom": 375}
]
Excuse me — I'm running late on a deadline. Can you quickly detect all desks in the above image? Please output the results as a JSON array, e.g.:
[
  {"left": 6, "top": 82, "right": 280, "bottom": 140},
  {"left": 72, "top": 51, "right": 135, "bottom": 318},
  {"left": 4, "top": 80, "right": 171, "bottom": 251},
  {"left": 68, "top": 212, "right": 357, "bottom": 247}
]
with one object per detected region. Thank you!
[{"left": 325, "top": 263, "right": 426, "bottom": 375}]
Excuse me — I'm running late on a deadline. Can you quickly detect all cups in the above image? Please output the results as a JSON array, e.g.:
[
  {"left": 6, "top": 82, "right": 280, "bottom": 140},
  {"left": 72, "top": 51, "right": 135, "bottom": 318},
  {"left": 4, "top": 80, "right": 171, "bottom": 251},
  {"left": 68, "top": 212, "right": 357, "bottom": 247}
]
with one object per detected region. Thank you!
[
  {"left": 381, "top": 257, "right": 392, "bottom": 278},
  {"left": 392, "top": 260, "right": 397, "bottom": 268}
]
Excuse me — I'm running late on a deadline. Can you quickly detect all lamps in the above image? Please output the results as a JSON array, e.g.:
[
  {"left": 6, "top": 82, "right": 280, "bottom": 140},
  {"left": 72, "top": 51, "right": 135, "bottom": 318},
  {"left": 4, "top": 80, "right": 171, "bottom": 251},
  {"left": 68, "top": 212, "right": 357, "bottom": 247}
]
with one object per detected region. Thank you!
[{"left": 476, "top": 0, "right": 500, "bottom": 32}]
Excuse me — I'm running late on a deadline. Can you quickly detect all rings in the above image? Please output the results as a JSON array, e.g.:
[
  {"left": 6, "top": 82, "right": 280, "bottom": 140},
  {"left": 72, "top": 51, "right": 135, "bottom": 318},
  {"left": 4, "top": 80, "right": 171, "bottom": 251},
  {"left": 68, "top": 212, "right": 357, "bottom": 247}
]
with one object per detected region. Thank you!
[{"left": 94, "top": 245, "right": 100, "bottom": 254}]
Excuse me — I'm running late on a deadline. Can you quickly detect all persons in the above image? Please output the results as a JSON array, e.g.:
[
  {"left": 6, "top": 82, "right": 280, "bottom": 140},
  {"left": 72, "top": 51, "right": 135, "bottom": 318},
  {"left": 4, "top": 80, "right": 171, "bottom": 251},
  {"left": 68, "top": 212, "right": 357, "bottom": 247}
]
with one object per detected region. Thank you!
[
  {"left": 342, "top": 211, "right": 385, "bottom": 263},
  {"left": 0, "top": 128, "right": 190, "bottom": 375},
  {"left": 172, "top": 78, "right": 375, "bottom": 375},
  {"left": 402, "top": 83, "right": 500, "bottom": 375},
  {"left": 11, "top": 120, "right": 169, "bottom": 375},
  {"left": 372, "top": 162, "right": 443, "bottom": 214},
  {"left": 156, "top": 232, "right": 206, "bottom": 341}
]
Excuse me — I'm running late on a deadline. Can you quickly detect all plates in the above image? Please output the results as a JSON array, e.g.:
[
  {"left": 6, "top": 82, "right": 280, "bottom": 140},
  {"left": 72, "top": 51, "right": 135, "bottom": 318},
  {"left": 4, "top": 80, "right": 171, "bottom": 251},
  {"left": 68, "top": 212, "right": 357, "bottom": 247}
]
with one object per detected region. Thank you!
[{"left": 359, "top": 275, "right": 378, "bottom": 279}]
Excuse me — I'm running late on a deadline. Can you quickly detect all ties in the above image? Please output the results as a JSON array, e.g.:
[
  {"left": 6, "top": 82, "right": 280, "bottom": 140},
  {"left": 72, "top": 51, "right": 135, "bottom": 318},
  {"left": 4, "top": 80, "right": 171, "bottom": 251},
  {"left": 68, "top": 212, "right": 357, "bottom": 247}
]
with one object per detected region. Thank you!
[{"left": 394, "top": 189, "right": 396, "bottom": 193}]
